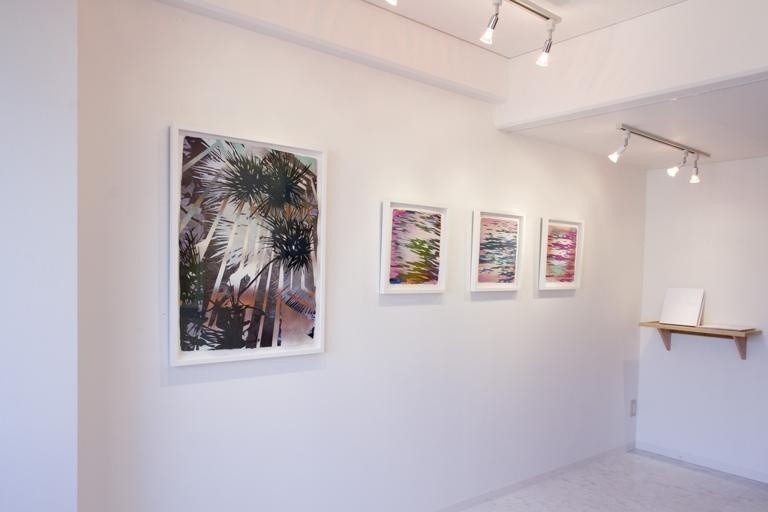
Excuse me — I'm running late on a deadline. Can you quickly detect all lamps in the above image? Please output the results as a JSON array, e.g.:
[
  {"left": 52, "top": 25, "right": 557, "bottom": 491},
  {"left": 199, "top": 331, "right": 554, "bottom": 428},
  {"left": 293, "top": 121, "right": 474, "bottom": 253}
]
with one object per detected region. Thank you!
[
  {"left": 478, "top": 0, "right": 562, "bottom": 69},
  {"left": 607, "top": 121, "right": 717, "bottom": 189}
]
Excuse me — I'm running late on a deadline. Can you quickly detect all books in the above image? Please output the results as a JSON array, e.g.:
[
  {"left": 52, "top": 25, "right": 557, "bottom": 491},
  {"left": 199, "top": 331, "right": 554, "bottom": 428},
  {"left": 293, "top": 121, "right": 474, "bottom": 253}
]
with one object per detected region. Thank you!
[
  {"left": 659, "top": 288, "right": 704, "bottom": 327},
  {"left": 706, "top": 325, "right": 754, "bottom": 332}
]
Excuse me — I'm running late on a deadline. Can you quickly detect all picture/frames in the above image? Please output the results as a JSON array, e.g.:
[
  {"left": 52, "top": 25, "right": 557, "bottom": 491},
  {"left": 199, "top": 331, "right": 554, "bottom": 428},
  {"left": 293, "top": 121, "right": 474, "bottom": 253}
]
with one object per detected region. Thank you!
[
  {"left": 537, "top": 215, "right": 583, "bottom": 292},
  {"left": 469, "top": 207, "right": 524, "bottom": 294},
  {"left": 376, "top": 198, "right": 449, "bottom": 297},
  {"left": 163, "top": 123, "right": 328, "bottom": 371}
]
